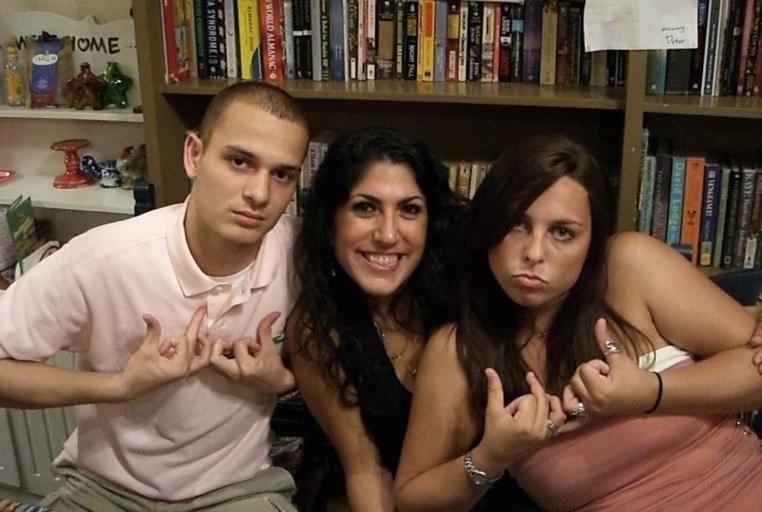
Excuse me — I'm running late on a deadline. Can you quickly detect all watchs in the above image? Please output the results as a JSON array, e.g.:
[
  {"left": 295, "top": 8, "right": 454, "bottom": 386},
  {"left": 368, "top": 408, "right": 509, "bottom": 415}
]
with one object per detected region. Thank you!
[{"left": 463, "top": 449, "right": 504, "bottom": 489}]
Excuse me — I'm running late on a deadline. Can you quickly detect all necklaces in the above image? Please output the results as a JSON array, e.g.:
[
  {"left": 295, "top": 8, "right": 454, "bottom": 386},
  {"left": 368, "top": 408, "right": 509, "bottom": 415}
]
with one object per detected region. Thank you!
[
  {"left": 374, "top": 319, "right": 418, "bottom": 376},
  {"left": 532, "top": 320, "right": 550, "bottom": 341}
]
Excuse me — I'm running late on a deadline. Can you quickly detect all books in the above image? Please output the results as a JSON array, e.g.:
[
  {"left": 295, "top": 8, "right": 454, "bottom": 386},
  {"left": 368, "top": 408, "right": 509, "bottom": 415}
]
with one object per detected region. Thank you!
[
  {"left": 158, "top": 1, "right": 628, "bottom": 89},
  {"left": 645, "top": 1, "right": 761, "bottom": 99},
  {"left": 633, "top": 128, "right": 762, "bottom": 270},
  {"left": 284, "top": 130, "right": 495, "bottom": 216}
]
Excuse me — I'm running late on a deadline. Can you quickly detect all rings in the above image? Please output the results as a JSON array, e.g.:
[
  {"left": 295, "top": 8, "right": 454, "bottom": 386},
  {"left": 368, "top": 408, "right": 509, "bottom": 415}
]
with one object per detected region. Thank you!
[
  {"left": 545, "top": 421, "right": 556, "bottom": 431},
  {"left": 603, "top": 339, "right": 620, "bottom": 356},
  {"left": 571, "top": 403, "right": 586, "bottom": 417}
]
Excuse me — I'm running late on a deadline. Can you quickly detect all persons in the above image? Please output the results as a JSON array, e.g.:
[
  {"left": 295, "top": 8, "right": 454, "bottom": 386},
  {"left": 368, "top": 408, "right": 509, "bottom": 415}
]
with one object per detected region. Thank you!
[
  {"left": 0, "top": 81, "right": 317, "bottom": 512},
  {"left": 394, "top": 136, "right": 762, "bottom": 511},
  {"left": 281, "top": 115, "right": 761, "bottom": 510}
]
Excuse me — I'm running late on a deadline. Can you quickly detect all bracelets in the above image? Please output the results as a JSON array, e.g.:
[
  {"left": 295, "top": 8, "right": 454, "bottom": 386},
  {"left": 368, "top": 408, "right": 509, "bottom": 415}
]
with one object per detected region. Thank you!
[
  {"left": 647, "top": 370, "right": 664, "bottom": 416},
  {"left": 278, "top": 390, "right": 299, "bottom": 403}
]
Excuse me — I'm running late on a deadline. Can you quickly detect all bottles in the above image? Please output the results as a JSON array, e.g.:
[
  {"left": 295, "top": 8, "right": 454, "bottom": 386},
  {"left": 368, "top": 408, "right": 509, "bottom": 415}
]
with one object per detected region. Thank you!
[{"left": 4, "top": 45, "right": 26, "bottom": 107}]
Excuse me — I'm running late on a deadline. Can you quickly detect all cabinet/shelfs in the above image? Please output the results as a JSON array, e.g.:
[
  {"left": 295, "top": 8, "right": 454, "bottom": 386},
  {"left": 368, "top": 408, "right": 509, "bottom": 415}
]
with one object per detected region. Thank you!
[
  {"left": 128, "top": 0, "right": 639, "bottom": 272},
  {"left": 631, "top": 0, "right": 762, "bottom": 318},
  {"left": 0, "top": 5, "right": 155, "bottom": 300},
  {"left": 0, "top": 343, "right": 84, "bottom": 502}
]
